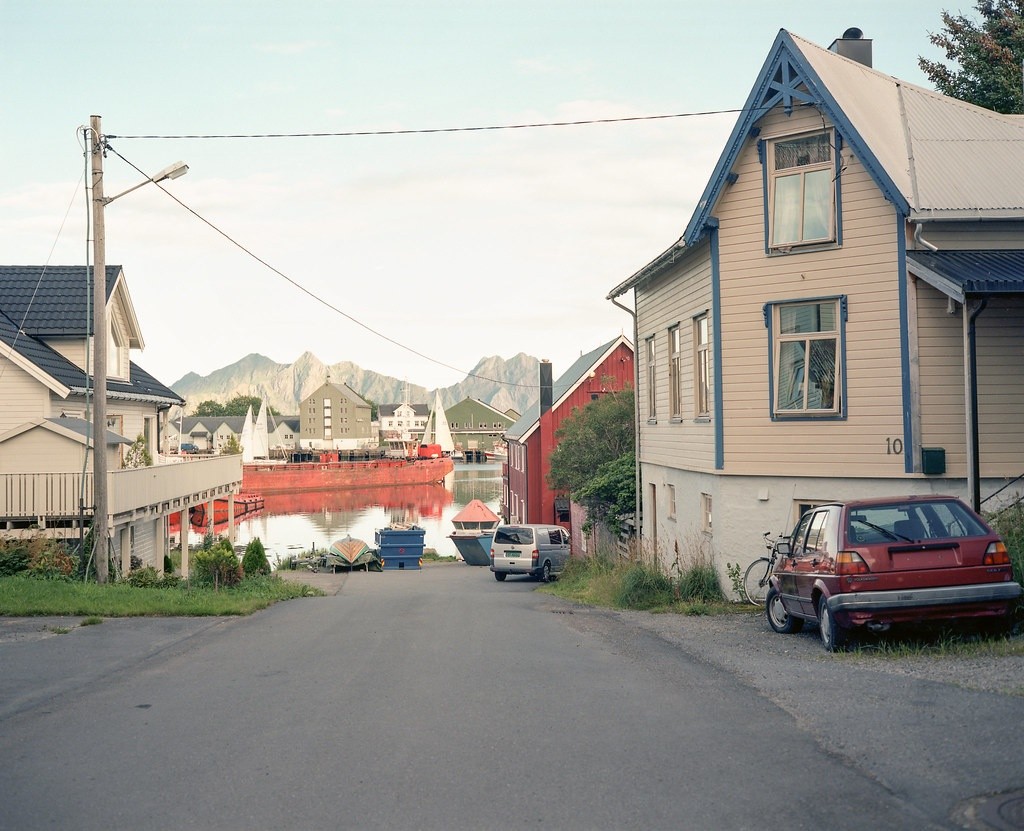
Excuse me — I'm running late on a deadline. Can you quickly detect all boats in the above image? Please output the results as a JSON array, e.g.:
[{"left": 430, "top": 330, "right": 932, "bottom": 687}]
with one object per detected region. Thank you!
[
  {"left": 238, "top": 438, "right": 454, "bottom": 493},
  {"left": 189, "top": 505, "right": 264, "bottom": 528},
  {"left": 194, "top": 494, "right": 264, "bottom": 513},
  {"left": 485, "top": 453, "right": 508, "bottom": 459}
]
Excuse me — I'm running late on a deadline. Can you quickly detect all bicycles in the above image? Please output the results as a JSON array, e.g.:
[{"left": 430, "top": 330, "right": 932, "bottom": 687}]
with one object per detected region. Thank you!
[{"left": 743, "top": 531, "right": 791, "bottom": 608}]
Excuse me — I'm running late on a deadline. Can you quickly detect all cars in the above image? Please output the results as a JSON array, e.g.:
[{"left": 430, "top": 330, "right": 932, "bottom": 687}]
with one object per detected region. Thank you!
[{"left": 766, "top": 494, "right": 1022, "bottom": 651}]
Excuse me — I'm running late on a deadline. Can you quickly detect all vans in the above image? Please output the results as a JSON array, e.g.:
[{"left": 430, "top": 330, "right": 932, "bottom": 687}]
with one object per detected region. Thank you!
[{"left": 489, "top": 523, "right": 571, "bottom": 583}]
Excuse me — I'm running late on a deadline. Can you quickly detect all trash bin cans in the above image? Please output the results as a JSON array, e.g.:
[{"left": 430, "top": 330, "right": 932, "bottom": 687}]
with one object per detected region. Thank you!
[{"left": 375, "top": 526, "right": 425, "bottom": 570}]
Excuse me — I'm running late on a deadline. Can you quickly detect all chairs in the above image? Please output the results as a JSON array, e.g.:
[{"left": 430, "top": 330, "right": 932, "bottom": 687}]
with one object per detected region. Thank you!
[{"left": 894, "top": 520, "right": 924, "bottom": 541}]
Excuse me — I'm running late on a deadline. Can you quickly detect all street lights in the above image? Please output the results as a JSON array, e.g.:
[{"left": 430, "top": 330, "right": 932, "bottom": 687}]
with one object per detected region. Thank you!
[
  {"left": 83, "top": 113, "right": 190, "bottom": 588},
  {"left": 163, "top": 409, "right": 181, "bottom": 456}
]
